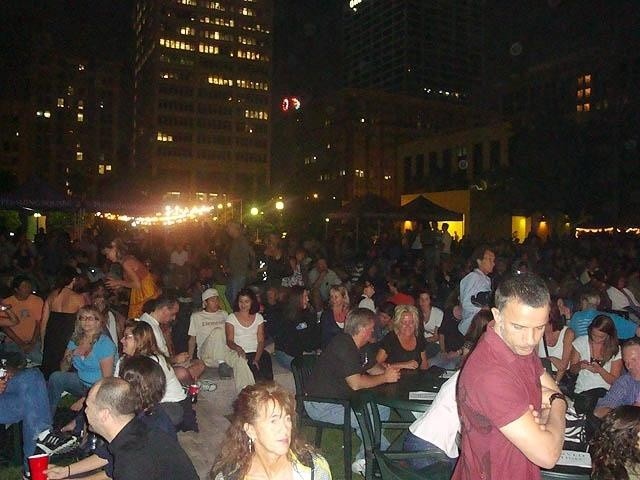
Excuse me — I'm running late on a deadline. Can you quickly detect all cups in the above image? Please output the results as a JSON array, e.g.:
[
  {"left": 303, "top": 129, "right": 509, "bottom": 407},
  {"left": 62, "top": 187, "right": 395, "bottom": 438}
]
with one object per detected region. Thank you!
[
  {"left": 189, "top": 384, "right": 199, "bottom": 394},
  {"left": 28, "top": 454, "right": 48, "bottom": 480}
]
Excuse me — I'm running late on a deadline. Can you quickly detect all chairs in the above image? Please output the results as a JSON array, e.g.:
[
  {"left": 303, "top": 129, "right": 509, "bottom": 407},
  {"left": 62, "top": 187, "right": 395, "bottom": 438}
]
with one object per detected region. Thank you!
[
  {"left": 352, "top": 388, "right": 448, "bottom": 478},
  {"left": 291, "top": 357, "right": 352, "bottom": 477}
]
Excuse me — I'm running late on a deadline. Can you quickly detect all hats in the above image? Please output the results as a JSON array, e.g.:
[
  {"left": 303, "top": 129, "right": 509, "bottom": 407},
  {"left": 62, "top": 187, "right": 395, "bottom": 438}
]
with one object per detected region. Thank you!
[{"left": 202, "top": 288, "right": 219, "bottom": 308}]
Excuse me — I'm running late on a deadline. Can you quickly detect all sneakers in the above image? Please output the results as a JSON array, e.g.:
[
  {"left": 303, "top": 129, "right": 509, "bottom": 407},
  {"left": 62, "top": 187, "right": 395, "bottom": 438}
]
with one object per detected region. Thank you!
[
  {"left": 35, "top": 433, "right": 76, "bottom": 455},
  {"left": 182, "top": 386, "right": 199, "bottom": 395},
  {"left": 24, "top": 471, "right": 31, "bottom": 480},
  {"left": 197, "top": 382, "right": 216, "bottom": 392},
  {"left": 351, "top": 459, "right": 365, "bottom": 479},
  {"left": 219, "top": 363, "right": 230, "bottom": 378}
]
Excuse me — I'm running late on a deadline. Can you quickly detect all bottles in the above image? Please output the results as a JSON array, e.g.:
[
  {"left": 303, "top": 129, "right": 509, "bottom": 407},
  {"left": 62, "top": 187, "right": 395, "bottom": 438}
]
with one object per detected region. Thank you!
[{"left": 188, "top": 376, "right": 199, "bottom": 405}]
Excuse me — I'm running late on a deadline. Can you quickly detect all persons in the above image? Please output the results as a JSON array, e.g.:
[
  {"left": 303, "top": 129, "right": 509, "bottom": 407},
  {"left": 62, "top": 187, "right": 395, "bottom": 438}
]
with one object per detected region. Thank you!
[
  {"left": 589, "top": 406, "right": 640, "bottom": 480},
  {"left": 570, "top": 316, "right": 622, "bottom": 416},
  {"left": 538, "top": 304, "right": 574, "bottom": 386},
  {"left": 570, "top": 294, "right": 640, "bottom": 342},
  {"left": 0, "top": 224, "right": 104, "bottom": 285},
  {"left": 0, "top": 302, "right": 19, "bottom": 343},
  {"left": 115, "top": 319, "right": 192, "bottom": 430},
  {"left": 3, "top": 278, "right": 44, "bottom": 362},
  {"left": 137, "top": 294, "right": 205, "bottom": 395},
  {"left": 84, "top": 377, "right": 200, "bottom": 480},
  {"left": 0, "top": 366, "right": 77, "bottom": 480},
  {"left": 48, "top": 305, "right": 116, "bottom": 425},
  {"left": 303, "top": 309, "right": 400, "bottom": 478},
  {"left": 378, "top": 302, "right": 395, "bottom": 336},
  {"left": 226, "top": 286, "right": 275, "bottom": 385},
  {"left": 321, "top": 287, "right": 352, "bottom": 337},
  {"left": 42, "top": 270, "right": 85, "bottom": 354},
  {"left": 257, "top": 221, "right": 464, "bottom": 307},
  {"left": 495, "top": 228, "right": 640, "bottom": 295},
  {"left": 595, "top": 341, "right": 640, "bottom": 417},
  {"left": 607, "top": 277, "right": 640, "bottom": 311},
  {"left": 103, "top": 239, "right": 162, "bottom": 323},
  {"left": 277, "top": 286, "right": 322, "bottom": 353},
  {"left": 402, "top": 309, "right": 493, "bottom": 477},
  {"left": 161, "top": 226, "right": 225, "bottom": 292},
  {"left": 189, "top": 288, "right": 255, "bottom": 393},
  {"left": 32, "top": 354, "right": 177, "bottom": 479},
  {"left": 450, "top": 272, "right": 568, "bottom": 480},
  {"left": 224, "top": 221, "right": 257, "bottom": 309},
  {"left": 419, "top": 293, "right": 444, "bottom": 356},
  {"left": 209, "top": 381, "right": 331, "bottom": 480},
  {"left": 460, "top": 246, "right": 496, "bottom": 336},
  {"left": 378, "top": 305, "right": 430, "bottom": 374}
]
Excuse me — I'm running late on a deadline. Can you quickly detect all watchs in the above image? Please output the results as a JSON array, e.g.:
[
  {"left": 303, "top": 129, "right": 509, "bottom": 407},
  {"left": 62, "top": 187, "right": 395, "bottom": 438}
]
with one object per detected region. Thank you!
[
  {"left": 1, "top": 306, "right": 9, "bottom": 311},
  {"left": 547, "top": 392, "right": 570, "bottom": 410}
]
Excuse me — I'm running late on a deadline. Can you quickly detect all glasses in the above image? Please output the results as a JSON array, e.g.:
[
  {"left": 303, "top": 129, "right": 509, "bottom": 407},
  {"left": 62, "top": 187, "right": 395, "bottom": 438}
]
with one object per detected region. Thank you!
[
  {"left": 124, "top": 334, "right": 134, "bottom": 340},
  {"left": 80, "top": 316, "right": 97, "bottom": 321}
]
